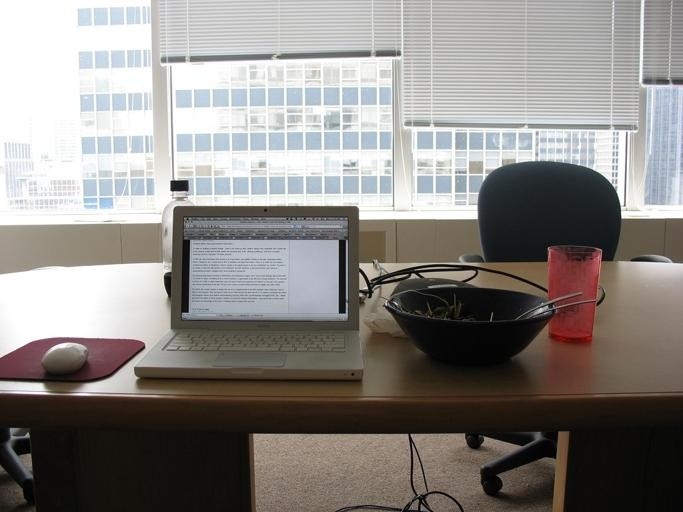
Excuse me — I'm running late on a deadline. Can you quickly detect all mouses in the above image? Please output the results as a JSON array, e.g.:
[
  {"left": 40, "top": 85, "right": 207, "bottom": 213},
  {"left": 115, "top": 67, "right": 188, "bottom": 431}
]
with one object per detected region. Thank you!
[{"left": 41, "top": 341, "right": 89, "bottom": 375}]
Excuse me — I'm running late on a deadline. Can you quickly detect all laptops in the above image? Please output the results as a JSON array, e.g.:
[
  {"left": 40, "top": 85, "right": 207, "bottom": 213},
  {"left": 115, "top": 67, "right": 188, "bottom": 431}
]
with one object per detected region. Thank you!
[{"left": 133, "top": 204, "right": 364, "bottom": 382}]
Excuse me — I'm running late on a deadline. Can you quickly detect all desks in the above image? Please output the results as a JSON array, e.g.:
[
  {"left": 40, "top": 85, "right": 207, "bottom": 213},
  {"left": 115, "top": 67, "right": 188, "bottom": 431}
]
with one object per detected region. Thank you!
[{"left": 0, "top": 261, "right": 681, "bottom": 511}]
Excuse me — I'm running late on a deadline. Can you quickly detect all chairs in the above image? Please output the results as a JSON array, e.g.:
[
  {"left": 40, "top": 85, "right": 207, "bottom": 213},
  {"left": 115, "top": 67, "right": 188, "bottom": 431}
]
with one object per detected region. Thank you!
[{"left": 455, "top": 161, "right": 676, "bottom": 495}]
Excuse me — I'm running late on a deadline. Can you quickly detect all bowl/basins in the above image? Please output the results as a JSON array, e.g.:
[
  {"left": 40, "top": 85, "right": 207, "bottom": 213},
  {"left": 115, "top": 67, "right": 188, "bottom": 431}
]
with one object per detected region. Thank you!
[{"left": 383, "top": 286, "right": 557, "bottom": 368}]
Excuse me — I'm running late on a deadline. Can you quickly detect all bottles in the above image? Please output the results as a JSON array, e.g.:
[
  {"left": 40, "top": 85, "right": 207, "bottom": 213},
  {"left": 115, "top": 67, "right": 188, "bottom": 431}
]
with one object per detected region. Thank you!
[{"left": 161, "top": 178, "right": 196, "bottom": 299}]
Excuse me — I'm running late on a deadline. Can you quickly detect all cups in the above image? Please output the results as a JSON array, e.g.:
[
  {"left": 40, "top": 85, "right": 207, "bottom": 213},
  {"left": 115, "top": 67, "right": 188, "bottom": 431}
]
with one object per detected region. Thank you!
[{"left": 546, "top": 244, "right": 603, "bottom": 344}]
